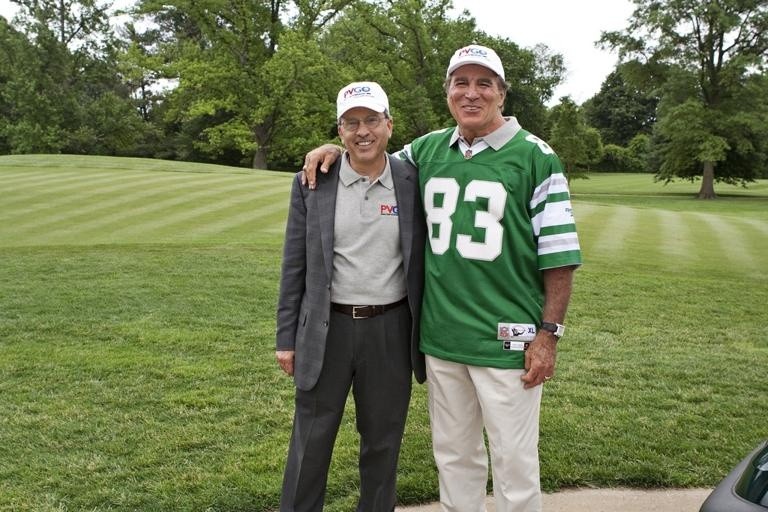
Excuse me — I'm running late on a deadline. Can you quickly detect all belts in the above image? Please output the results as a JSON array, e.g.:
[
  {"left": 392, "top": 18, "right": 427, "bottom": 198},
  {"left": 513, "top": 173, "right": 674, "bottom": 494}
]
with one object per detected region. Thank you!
[{"left": 330, "top": 295, "right": 408, "bottom": 319}]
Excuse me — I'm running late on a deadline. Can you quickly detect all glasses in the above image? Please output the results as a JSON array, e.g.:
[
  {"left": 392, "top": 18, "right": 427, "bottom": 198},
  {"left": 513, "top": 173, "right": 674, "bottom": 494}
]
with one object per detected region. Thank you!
[{"left": 336, "top": 115, "right": 383, "bottom": 130}]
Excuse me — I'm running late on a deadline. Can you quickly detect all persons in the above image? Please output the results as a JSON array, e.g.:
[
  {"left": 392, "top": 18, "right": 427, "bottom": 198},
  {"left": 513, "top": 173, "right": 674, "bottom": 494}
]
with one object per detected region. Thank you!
[
  {"left": 300, "top": 43, "right": 583, "bottom": 512},
  {"left": 276, "top": 80, "right": 428, "bottom": 512}
]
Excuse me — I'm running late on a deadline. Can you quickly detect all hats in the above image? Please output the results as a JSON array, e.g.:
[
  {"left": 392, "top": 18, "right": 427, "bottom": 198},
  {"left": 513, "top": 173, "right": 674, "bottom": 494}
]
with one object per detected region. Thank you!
[
  {"left": 335, "top": 81, "right": 390, "bottom": 120},
  {"left": 446, "top": 44, "right": 506, "bottom": 81}
]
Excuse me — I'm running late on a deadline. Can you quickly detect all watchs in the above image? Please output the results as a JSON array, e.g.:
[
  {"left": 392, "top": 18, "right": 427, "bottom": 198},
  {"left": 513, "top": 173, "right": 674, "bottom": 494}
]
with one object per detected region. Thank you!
[{"left": 541, "top": 319, "right": 566, "bottom": 338}]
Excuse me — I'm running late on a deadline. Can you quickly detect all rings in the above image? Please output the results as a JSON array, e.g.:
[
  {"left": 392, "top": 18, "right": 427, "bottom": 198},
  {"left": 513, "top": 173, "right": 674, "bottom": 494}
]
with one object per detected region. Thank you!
[{"left": 543, "top": 375, "right": 551, "bottom": 380}]
